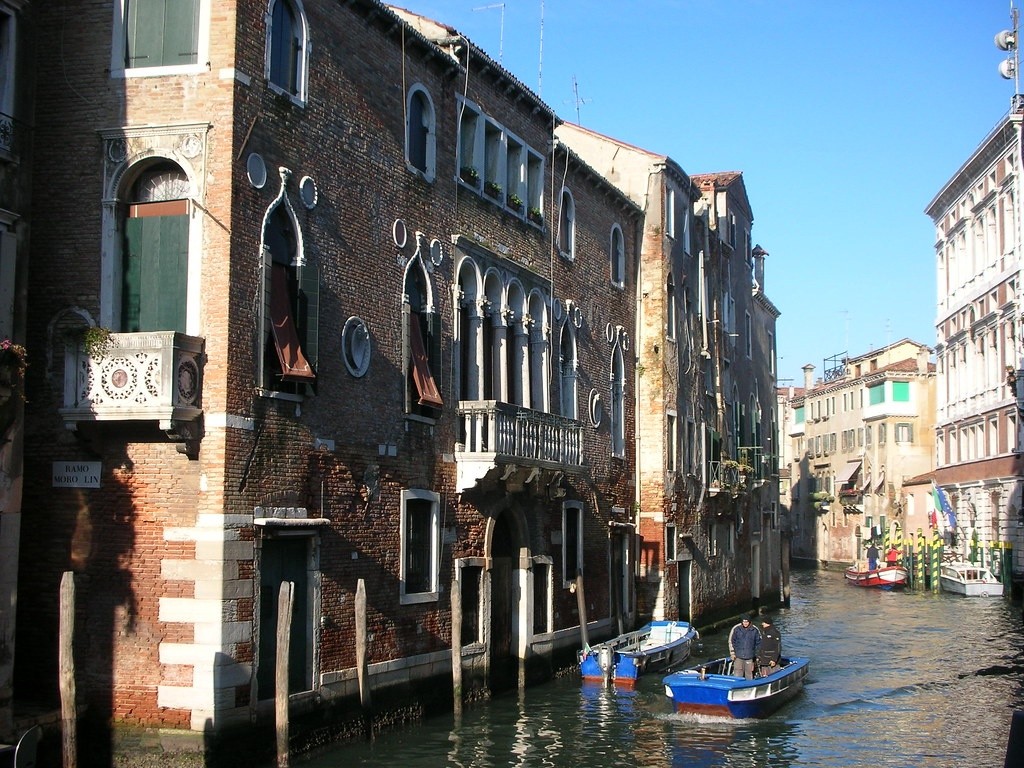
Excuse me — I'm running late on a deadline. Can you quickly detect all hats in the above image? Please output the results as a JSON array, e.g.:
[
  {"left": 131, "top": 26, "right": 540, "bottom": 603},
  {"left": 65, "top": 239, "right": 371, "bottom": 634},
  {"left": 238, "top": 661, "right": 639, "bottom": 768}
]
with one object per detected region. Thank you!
[
  {"left": 741, "top": 614, "right": 751, "bottom": 623},
  {"left": 763, "top": 617, "right": 773, "bottom": 624}
]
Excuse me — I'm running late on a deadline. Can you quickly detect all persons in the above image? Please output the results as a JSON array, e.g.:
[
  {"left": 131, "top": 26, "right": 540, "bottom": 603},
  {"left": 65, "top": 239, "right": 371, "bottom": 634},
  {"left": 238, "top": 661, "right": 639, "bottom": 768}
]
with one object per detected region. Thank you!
[
  {"left": 728, "top": 618, "right": 762, "bottom": 681},
  {"left": 867, "top": 543, "right": 879, "bottom": 571},
  {"left": 887, "top": 544, "right": 901, "bottom": 567},
  {"left": 759, "top": 617, "right": 782, "bottom": 676}
]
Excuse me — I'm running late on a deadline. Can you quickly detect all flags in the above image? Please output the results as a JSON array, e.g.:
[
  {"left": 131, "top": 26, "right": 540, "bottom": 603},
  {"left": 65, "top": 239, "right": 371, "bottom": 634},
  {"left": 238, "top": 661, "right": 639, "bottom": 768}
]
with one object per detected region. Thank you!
[
  {"left": 932, "top": 482, "right": 944, "bottom": 541},
  {"left": 935, "top": 483, "right": 957, "bottom": 535}
]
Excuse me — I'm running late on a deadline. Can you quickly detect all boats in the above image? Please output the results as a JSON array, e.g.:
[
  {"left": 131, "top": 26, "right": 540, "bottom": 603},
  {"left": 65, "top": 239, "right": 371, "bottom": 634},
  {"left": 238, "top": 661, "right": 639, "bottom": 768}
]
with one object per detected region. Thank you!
[
  {"left": 844, "top": 564, "right": 909, "bottom": 591},
  {"left": 662, "top": 654, "right": 814, "bottom": 720},
  {"left": 939, "top": 565, "right": 1005, "bottom": 598},
  {"left": 581, "top": 620, "right": 701, "bottom": 687}
]
